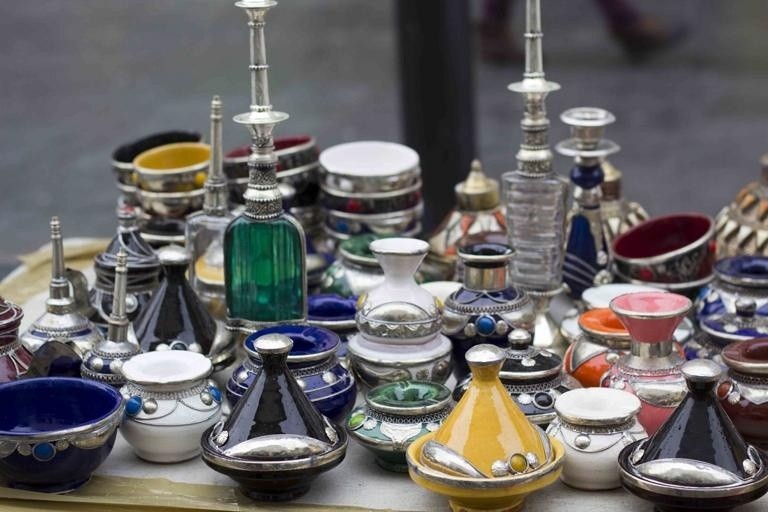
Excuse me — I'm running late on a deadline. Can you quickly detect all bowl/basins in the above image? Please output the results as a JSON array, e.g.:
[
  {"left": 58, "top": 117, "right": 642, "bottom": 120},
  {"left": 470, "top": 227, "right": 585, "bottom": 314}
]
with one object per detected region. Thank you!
[
  {"left": 611, "top": 212, "right": 716, "bottom": 293},
  {"left": 112, "top": 134, "right": 316, "bottom": 220},
  {"left": 1, "top": 376, "right": 121, "bottom": 495},
  {"left": 316, "top": 141, "right": 424, "bottom": 246}
]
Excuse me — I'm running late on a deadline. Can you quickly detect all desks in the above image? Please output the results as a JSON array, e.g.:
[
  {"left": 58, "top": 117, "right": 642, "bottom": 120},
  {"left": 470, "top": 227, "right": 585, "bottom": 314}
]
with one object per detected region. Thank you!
[{"left": 2, "top": 232, "right": 768, "bottom": 512}]
